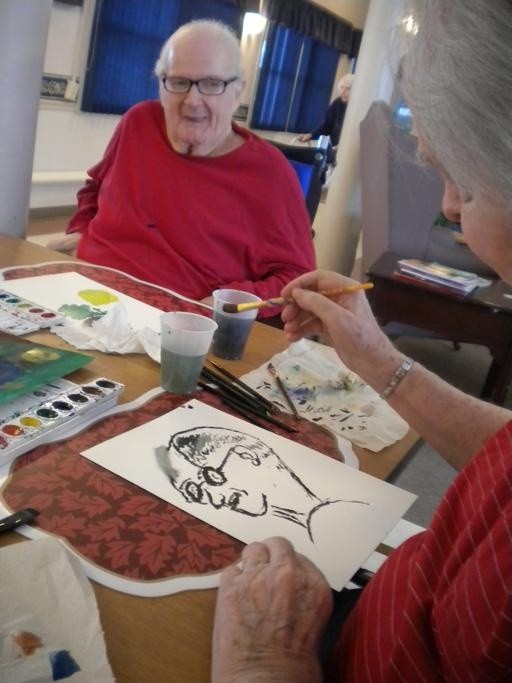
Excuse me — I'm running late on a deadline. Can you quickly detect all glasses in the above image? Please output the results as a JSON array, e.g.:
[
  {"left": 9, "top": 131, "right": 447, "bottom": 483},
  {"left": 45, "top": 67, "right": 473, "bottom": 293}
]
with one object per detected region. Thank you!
[{"left": 158, "top": 70, "right": 239, "bottom": 97}]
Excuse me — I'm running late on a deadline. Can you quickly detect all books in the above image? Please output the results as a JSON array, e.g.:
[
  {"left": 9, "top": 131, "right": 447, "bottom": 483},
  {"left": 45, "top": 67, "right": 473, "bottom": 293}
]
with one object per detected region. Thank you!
[{"left": 391, "top": 255, "right": 495, "bottom": 303}]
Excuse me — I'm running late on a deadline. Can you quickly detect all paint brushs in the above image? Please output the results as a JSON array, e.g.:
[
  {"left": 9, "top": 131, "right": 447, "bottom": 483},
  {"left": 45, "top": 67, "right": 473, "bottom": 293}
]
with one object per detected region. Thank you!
[
  {"left": 223, "top": 283, "right": 374, "bottom": 313},
  {"left": 0, "top": 508, "right": 40, "bottom": 532},
  {"left": 197, "top": 359, "right": 300, "bottom": 434}
]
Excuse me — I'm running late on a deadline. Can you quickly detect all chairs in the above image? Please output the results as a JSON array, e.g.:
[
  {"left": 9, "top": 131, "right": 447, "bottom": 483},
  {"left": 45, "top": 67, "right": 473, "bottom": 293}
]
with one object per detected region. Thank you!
[{"left": 266, "top": 139, "right": 329, "bottom": 232}]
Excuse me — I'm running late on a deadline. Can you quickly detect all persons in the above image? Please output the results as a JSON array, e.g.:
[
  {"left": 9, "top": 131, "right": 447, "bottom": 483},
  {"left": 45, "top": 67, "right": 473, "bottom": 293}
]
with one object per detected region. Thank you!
[
  {"left": 45, "top": 17, "right": 317, "bottom": 320},
  {"left": 208, "top": 1, "right": 512, "bottom": 683},
  {"left": 296, "top": 71, "right": 356, "bottom": 182}
]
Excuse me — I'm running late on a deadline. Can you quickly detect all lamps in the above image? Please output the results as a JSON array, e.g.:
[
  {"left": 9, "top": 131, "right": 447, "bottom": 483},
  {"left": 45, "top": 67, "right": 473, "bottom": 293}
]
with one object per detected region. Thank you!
[{"left": 242, "top": 10, "right": 268, "bottom": 35}]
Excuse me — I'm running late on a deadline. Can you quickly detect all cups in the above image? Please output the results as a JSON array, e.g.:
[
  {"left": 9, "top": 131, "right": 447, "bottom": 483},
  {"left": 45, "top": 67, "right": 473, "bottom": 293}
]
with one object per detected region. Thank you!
[
  {"left": 212, "top": 288, "right": 261, "bottom": 359},
  {"left": 159, "top": 310, "right": 218, "bottom": 395}
]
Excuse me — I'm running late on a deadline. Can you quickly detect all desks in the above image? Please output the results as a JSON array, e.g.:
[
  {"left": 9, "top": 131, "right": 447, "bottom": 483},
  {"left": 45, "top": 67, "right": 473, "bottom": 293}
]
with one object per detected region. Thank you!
[{"left": 0, "top": 231, "right": 423, "bottom": 683}]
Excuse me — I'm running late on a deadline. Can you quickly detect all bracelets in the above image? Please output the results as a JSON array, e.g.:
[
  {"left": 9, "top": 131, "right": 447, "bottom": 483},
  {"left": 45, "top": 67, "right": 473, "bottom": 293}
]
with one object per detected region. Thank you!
[{"left": 380, "top": 356, "right": 414, "bottom": 400}]
